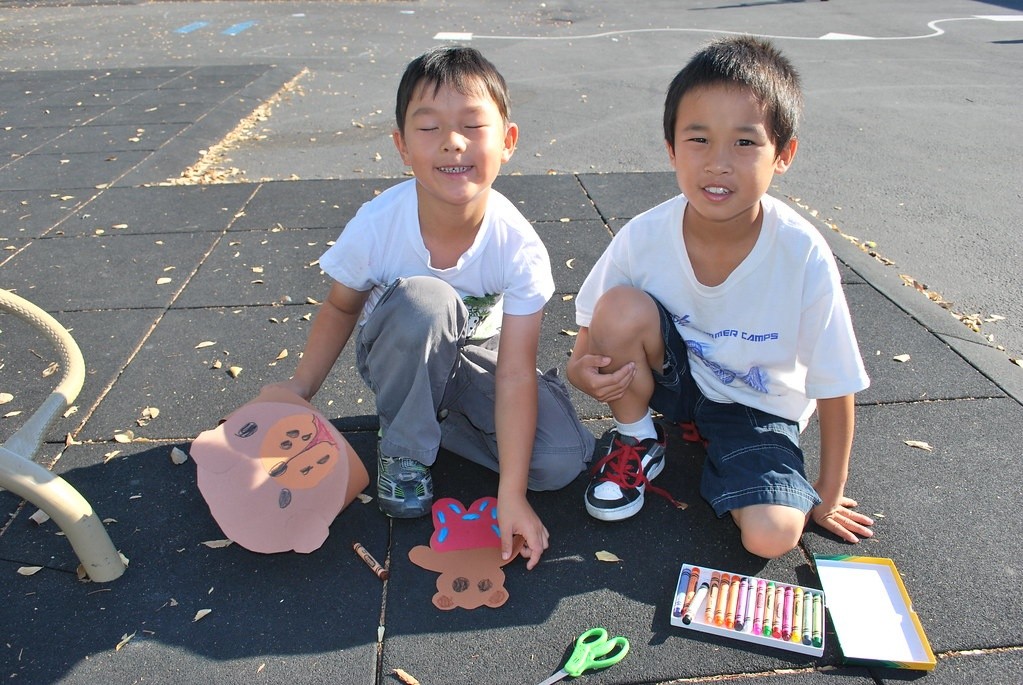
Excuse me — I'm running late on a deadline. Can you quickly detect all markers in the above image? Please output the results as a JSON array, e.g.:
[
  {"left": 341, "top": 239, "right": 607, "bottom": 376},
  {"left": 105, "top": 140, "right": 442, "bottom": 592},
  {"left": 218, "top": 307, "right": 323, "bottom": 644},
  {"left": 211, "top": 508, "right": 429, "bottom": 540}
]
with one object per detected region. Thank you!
[
  {"left": 352, "top": 541, "right": 388, "bottom": 580},
  {"left": 669, "top": 562, "right": 826, "bottom": 658}
]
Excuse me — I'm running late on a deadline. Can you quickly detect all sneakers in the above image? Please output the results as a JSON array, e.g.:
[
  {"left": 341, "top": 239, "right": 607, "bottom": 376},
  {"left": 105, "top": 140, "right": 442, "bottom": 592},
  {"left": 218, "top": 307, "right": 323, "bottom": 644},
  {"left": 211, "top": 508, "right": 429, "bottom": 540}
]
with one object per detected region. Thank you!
[
  {"left": 584, "top": 422, "right": 688, "bottom": 520},
  {"left": 376, "top": 426, "right": 434, "bottom": 518},
  {"left": 681, "top": 419, "right": 709, "bottom": 452}
]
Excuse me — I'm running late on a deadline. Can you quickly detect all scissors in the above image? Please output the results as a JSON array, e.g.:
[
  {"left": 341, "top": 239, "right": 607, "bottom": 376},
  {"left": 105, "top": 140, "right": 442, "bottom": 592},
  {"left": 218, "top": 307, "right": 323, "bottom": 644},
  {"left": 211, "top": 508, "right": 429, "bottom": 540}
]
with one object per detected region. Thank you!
[{"left": 537, "top": 627, "right": 630, "bottom": 685}]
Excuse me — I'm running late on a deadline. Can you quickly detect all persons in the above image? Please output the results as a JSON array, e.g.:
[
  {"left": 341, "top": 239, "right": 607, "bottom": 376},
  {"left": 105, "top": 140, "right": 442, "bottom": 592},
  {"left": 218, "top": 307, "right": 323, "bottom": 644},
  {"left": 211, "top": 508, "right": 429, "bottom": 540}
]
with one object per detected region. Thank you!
[
  {"left": 259, "top": 43, "right": 597, "bottom": 572},
  {"left": 565, "top": 34, "right": 875, "bottom": 560}
]
